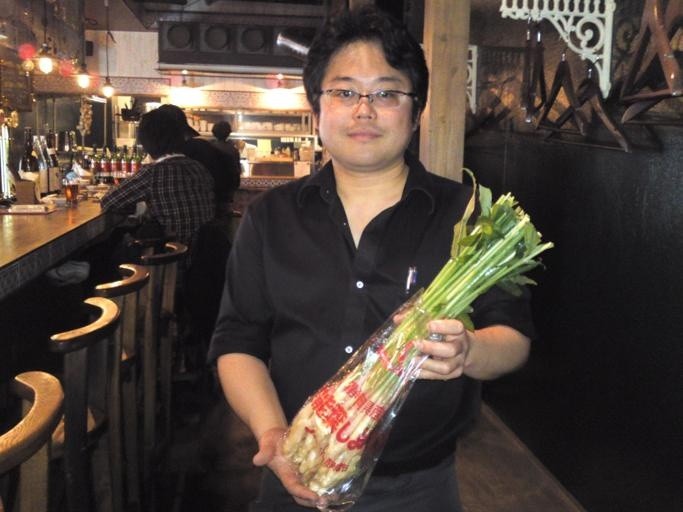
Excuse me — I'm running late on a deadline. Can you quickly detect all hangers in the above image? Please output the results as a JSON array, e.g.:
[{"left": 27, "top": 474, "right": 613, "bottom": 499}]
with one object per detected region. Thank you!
[
  {"left": 526, "top": 15, "right": 546, "bottom": 122},
  {"left": 536, "top": 34, "right": 593, "bottom": 139},
  {"left": 621, "top": 87, "right": 667, "bottom": 124},
  {"left": 544, "top": 57, "right": 631, "bottom": 153},
  {"left": 519, "top": 14, "right": 530, "bottom": 111},
  {"left": 619, "top": 0, "right": 683, "bottom": 105},
  {"left": 632, "top": 0, "right": 683, "bottom": 91}
]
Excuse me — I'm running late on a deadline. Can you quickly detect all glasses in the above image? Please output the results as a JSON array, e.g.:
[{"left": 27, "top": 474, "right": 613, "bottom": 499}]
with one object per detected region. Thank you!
[{"left": 318, "top": 88, "right": 416, "bottom": 106}]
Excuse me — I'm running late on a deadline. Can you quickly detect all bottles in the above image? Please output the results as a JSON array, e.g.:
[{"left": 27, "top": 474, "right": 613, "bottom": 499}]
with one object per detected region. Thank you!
[
  {"left": 109, "top": 144, "right": 119, "bottom": 177},
  {"left": 46, "top": 137, "right": 62, "bottom": 193},
  {"left": 33, "top": 133, "right": 48, "bottom": 196},
  {"left": 19, "top": 126, "right": 40, "bottom": 202},
  {"left": 39, "top": 136, "right": 55, "bottom": 194},
  {"left": 89, "top": 143, "right": 99, "bottom": 176},
  {"left": 129, "top": 145, "right": 138, "bottom": 177},
  {"left": 99, "top": 144, "right": 109, "bottom": 177},
  {"left": 119, "top": 145, "right": 130, "bottom": 177}
]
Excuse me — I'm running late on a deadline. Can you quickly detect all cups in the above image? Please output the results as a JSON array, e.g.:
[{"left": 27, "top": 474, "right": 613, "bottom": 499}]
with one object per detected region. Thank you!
[{"left": 61, "top": 182, "right": 79, "bottom": 207}]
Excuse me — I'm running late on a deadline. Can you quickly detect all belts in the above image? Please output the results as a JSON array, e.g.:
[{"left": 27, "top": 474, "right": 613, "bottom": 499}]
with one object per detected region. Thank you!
[{"left": 371, "top": 437, "right": 457, "bottom": 477}]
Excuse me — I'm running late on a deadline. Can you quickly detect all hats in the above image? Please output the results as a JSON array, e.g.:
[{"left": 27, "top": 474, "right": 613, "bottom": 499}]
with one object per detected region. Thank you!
[{"left": 159, "top": 104, "right": 200, "bottom": 136}]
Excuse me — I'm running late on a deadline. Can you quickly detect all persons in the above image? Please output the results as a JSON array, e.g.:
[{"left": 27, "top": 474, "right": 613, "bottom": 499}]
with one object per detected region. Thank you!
[
  {"left": 208, "top": 11, "right": 540, "bottom": 506},
  {"left": 206, "top": 119, "right": 240, "bottom": 247},
  {"left": 100, "top": 107, "right": 232, "bottom": 386}
]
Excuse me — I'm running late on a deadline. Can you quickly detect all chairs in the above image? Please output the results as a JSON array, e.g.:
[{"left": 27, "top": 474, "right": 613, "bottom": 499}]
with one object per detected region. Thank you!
[
  {"left": 93, "top": 262, "right": 151, "bottom": 508},
  {"left": 0, "top": 371, "right": 64, "bottom": 512},
  {"left": 129, "top": 236, "right": 189, "bottom": 426},
  {"left": 49, "top": 296, "right": 122, "bottom": 511}
]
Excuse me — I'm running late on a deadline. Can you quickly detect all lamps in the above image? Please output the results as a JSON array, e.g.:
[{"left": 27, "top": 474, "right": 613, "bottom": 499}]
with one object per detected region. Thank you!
[
  {"left": 76, "top": 3, "right": 90, "bottom": 89},
  {"left": 39, "top": 2, "right": 53, "bottom": 75},
  {"left": 100, "top": 1, "right": 116, "bottom": 98}
]
